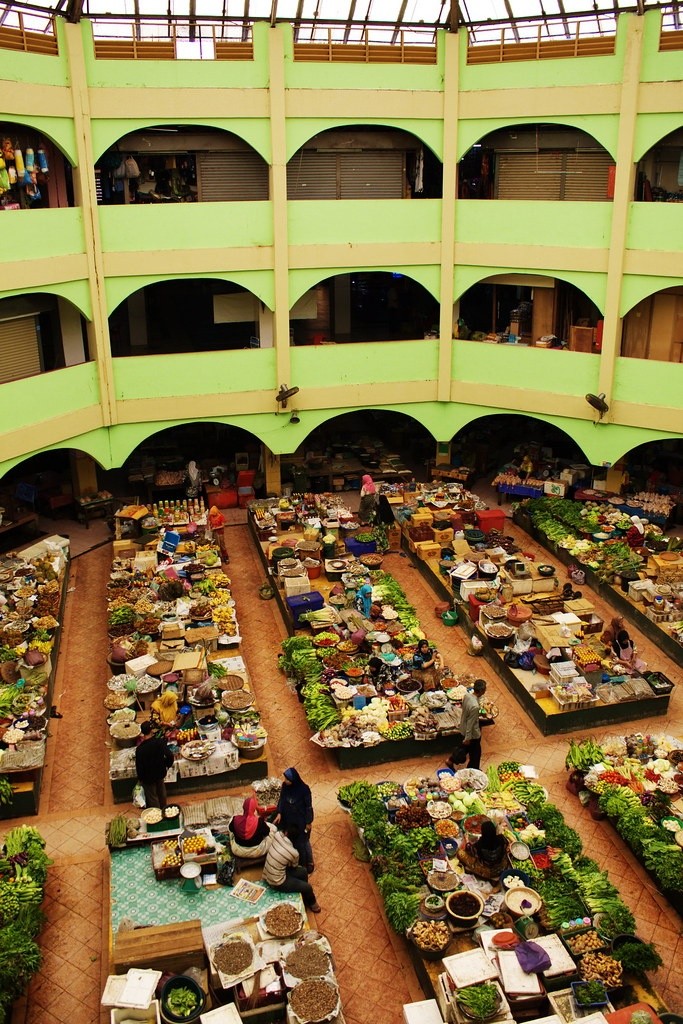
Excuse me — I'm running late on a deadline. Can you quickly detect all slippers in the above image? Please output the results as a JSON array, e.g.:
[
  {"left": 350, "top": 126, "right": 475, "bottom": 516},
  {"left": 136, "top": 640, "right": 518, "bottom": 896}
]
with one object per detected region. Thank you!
[{"left": 445, "top": 759, "right": 456, "bottom": 772}]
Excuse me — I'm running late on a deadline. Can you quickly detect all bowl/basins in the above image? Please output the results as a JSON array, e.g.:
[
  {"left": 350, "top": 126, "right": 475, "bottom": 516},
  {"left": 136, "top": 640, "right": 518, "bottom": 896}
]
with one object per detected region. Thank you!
[
  {"left": 160, "top": 975, "right": 207, "bottom": 1024},
  {"left": 411, "top": 919, "right": 452, "bottom": 959},
  {"left": 163, "top": 804, "right": 181, "bottom": 820},
  {"left": 538, "top": 565, "right": 555, "bottom": 577},
  {"left": 505, "top": 887, "right": 541, "bottom": 916},
  {"left": 661, "top": 816, "right": 683, "bottom": 832},
  {"left": 478, "top": 562, "right": 500, "bottom": 579},
  {"left": 501, "top": 869, "right": 529, "bottom": 892},
  {"left": 446, "top": 891, "right": 485, "bottom": 926}
]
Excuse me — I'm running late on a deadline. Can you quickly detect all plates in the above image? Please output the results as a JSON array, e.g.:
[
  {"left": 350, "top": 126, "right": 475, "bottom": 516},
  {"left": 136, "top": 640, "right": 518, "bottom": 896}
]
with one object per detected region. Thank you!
[{"left": 141, "top": 808, "right": 163, "bottom": 824}]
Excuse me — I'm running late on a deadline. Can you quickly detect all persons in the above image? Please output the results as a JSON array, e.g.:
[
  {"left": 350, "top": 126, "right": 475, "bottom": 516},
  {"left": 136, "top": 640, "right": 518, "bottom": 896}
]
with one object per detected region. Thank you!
[
  {"left": 272, "top": 768, "right": 315, "bottom": 875},
  {"left": 412, "top": 639, "right": 444, "bottom": 692},
  {"left": 358, "top": 475, "right": 375, "bottom": 527},
  {"left": 457, "top": 821, "right": 510, "bottom": 878},
  {"left": 611, "top": 630, "right": 649, "bottom": 675},
  {"left": 363, "top": 657, "right": 395, "bottom": 692},
  {"left": 142, "top": 453, "right": 157, "bottom": 504},
  {"left": 135, "top": 720, "right": 174, "bottom": 813},
  {"left": 227, "top": 797, "right": 277, "bottom": 858},
  {"left": 354, "top": 585, "right": 372, "bottom": 620},
  {"left": 445, "top": 680, "right": 487, "bottom": 772},
  {"left": 645, "top": 461, "right": 683, "bottom": 494},
  {"left": 261, "top": 820, "right": 321, "bottom": 914},
  {"left": 516, "top": 455, "right": 536, "bottom": 482},
  {"left": 369, "top": 495, "right": 395, "bottom": 556},
  {"left": 600, "top": 615, "right": 626, "bottom": 656},
  {"left": 181, "top": 461, "right": 202, "bottom": 500}
]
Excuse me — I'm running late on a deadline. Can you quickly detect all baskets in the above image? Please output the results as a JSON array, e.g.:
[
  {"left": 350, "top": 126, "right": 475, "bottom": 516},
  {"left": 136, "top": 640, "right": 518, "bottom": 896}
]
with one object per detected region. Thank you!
[
  {"left": 577, "top": 613, "right": 604, "bottom": 635},
  {"left": 151, "top": 839, "right": 183, "bottom": 881},
  {"left": 643, "top": 672, "right": 674, "bottom": 695}
]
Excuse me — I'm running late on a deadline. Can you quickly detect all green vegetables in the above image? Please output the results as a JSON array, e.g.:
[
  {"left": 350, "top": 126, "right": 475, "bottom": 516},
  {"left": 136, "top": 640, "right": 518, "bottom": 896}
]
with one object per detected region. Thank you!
[{"left": 0, "top": 495, "right": 683, "bottom": 1023}]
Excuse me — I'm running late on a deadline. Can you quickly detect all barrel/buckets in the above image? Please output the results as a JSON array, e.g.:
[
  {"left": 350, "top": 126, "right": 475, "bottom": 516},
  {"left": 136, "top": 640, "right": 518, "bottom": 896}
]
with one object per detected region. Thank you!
[{"left": 441, "top": 611, "right": 457, "bottom": 626}]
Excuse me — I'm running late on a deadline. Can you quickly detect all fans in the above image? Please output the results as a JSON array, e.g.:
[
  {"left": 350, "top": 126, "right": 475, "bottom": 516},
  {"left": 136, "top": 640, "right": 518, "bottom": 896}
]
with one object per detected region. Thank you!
[
  {"left": 275, "top": 384, "right": 299, "bottom": 408},
  {"left": 585, "top": 392, "right": 609, "bottom": 419}
]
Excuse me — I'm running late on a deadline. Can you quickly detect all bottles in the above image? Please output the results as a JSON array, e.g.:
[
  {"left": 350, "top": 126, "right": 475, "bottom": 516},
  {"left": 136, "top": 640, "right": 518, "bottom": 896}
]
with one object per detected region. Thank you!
[
  {"left": 152, "top": 504, "right": 158, "bottom": 518},
  {"left": 158, "top": 501, "right": 164, "bottom": 515},
  {"left": 194, "top": 499, "right": 199, "bottom": 514},
  {"left": 165, "top": 500, "right": 169, "bottom": 514},
  {"left": 176, "top": 500, "right": 181, "bottom": 510},
  {"left": 182, "top": 500, "right": 187, "bottom": 512},
  {"left": 200, "top": 500, "right": 205, "bottom": 514},
  {"left": 170, "top": 501, "right": 175, "bottom": 513},
  {"left": 188, "top": 499, "right": 194, "bottom": 515}
]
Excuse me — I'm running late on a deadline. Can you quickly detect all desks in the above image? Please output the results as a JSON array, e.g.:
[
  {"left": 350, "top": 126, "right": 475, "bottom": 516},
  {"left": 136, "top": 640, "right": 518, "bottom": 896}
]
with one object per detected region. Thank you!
[
  {"left": 74, "top": 493, "right": 115, "bottom": 530},
  {"left": 498, "top": 483, "right": 543, "bottom": 506}
]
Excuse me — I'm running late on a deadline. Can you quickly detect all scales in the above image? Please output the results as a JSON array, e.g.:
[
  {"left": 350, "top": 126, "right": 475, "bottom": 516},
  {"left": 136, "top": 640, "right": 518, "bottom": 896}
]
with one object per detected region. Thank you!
[
  {"left": 188, "top": 695, "right": 219, "bottom": 709},
  {"left": 415, "top": 496, "right": 425, "bottom": 507},
  {"left": 208, "top": 472, "right": 220, "bottom": 486},
  {"left": 280, "top": 504, "right": 293, "bottom": 510},
  {"left": 184, "top": 564, "right": 204, "bottom": 580},
  {"left": 180, "top": 860, "right": 204, "bottom": 892},
  {"left": 510, "top": 561, "right": 527, "bottom": 576},
  {"left": 375, "top": 633, "right": 393, "bottom": 654},
  {"left": 516, "top": 906, "right": 539, "bottom": 940},
  {"left": 543, "top": 464, "right": 553, "bottom": 477}
]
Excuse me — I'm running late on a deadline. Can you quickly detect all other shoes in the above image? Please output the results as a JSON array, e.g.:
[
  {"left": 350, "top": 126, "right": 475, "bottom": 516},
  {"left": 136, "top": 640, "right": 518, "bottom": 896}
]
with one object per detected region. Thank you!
[
  {"left": 309, "top": 902, "right": 321, "bottom": 913},
  {"left": 307, "top": 863, "right": 314, "bottom": 874}
]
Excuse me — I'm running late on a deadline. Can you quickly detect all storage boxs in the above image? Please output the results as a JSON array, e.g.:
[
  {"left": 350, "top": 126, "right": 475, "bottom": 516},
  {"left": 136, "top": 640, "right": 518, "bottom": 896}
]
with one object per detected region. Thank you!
[
  {"left": 476, "top": 510, "right": 506, "bottom": 533},
  {"left": 641, "top": 672, "right": 675, "bottom": 695},
  {"left": 503, "top": 570, "right": 532, "bottom": 594},
  {"left": 460, "top": 581, "right": 487, "bottom": 602},
  {"left": 402, "top": 492, "right": 456, "bottom": 561},
  {"left": 125, "top": 623, "right": 219, "bottom": 701},
  {"left": 577, "top": 613, "right": 604, "bottom": 634},
  {"left": 280, "top": 518, "right": 341, "bottom": 629},
  {"left": 526, "top": 562, "right": 554, "bottom": 591},
  {"left": 549, "top": 663, "right": 603, "bottom": 711},
  {"left": 544, "top": 477, "right": 569, "bottom": 496},
  {"left": 628, "top": 579, "right": 652, "bottom": 602},
  {"left": 560, "top": 469, "right": 579, "bottom": 486},
  {"left": 17, "top": 652, "right": 53, "bottom": 684}
]
